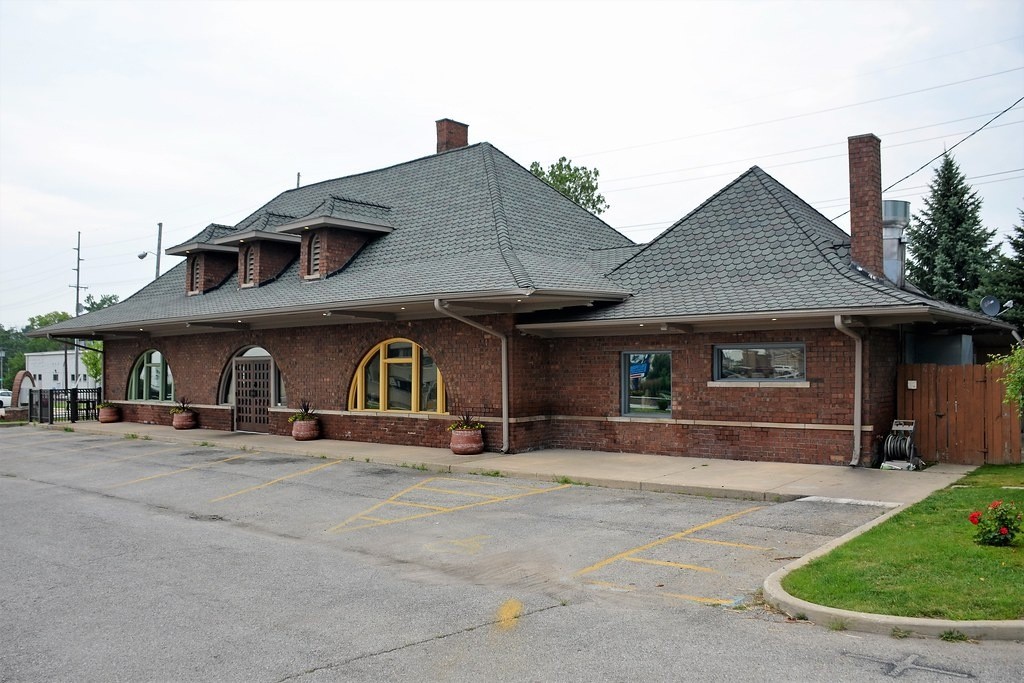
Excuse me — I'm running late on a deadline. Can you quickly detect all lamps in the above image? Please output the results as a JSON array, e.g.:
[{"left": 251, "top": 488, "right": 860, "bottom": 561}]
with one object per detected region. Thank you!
[{"left": 1002, "top": 299, "right": 1014, "bottom": 309}]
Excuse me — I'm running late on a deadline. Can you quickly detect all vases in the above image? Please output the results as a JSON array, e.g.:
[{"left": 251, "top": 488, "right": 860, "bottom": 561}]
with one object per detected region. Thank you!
[
  {"left": 291, "top": 419, "right": 321, "bottom": 442},
  {"left": 98, "top": 407, "right": 118, "bottom": 423},
  {"left": 449, "top": 429, "right": 484, "bottom": 455},
  {"left": 172, "top": 412, "right": 198, "bottom": 430}
]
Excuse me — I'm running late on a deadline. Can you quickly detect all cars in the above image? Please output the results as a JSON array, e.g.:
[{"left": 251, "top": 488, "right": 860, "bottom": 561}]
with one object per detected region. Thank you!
[{"left": 1, "top": 390, "right": 13, "bottom": 408}]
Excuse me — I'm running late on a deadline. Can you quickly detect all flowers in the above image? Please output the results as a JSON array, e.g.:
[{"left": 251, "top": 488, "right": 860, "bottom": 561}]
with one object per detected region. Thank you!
[
  {"left": 446, "top": 411, "right": 485, "bottom": 432},
  {"left": 968, "top": 500, "right": 1024, "bottom": 546},
  {"left": 287, "top": 397, "right": 321, "bottom": 423},
  {"left": 168, "top": 397, "right": 201, "bottom": 415},
  {"left": 96, "top": 401, "right": 117, "bottom": 409}
]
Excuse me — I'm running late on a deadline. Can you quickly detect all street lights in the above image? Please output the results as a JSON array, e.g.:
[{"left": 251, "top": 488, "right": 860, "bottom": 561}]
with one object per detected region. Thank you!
[{"left": 139, "top": 223, "right": 163, "bottom": 279}]
